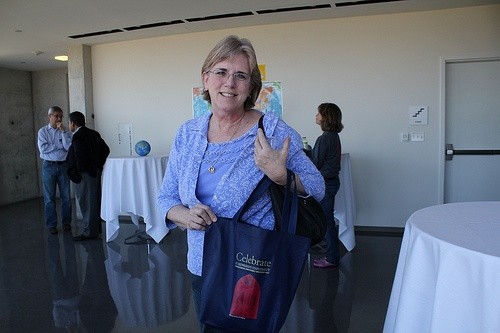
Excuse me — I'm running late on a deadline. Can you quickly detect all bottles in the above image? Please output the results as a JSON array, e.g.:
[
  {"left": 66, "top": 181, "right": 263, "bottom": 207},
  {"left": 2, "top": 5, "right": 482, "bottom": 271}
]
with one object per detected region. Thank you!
[{"left": 302, "top": 137, "right": 309, "bottom": 150}]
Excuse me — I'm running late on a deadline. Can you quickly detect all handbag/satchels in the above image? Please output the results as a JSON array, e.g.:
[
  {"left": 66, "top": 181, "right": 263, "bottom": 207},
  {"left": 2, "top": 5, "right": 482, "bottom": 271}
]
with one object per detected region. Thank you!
[{"left": 196, "top": 167, "right": 327, "bottom": 333}]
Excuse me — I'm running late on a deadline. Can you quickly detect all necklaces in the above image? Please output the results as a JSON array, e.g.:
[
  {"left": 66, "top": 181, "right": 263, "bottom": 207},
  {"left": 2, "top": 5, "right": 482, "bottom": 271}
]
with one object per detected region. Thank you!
[{"left": 207, "top": 110, "right": 246, "bottom": 174}]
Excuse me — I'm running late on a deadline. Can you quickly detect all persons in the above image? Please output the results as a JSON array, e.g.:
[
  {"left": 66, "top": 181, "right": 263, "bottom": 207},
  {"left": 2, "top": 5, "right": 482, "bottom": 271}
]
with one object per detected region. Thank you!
[
  {"left": 156, "top": 35, "right": 326, "bottom": 333},
  {"left": 48, "top": 231, "right": 119, "bottom": 333},
  {"left": 65, "top": 110, "right": 111, "bottom": 241},
  {"left": 38, "top": 107, "right": 74, "bottom": 233},
  {"left": 310, "top": 104, "right": 345, "bottom": 269}
]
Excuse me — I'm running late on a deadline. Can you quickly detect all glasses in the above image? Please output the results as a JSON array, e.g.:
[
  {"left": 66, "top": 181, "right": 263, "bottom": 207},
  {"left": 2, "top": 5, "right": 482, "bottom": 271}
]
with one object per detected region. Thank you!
[
  {"left": 206, "top": 68, "right": 250, "bottom": 82},
  {"left": 52, "top": 114, "right": 64, "bottom": 118}
]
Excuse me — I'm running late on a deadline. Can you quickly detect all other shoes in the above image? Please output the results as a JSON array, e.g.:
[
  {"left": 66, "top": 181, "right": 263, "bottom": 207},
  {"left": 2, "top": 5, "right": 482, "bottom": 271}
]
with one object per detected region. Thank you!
[
  {"left": 49, "top": 228, "right": 58, "bottom": 234},
  {"left": 312, "top": 256, "right": 339, "bottom": 267},
  {"left": 73, "top": 233, "right": 99, "bottom": 241},
  {"left": 63, "top": 224, "right": 72, "bottom": 230}
]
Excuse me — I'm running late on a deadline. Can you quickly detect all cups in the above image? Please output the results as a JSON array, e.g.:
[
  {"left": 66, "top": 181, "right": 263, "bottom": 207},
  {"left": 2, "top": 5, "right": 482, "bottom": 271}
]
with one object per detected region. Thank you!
[{"left": 265, "top": 136, "right": 283, "bottom": 151}]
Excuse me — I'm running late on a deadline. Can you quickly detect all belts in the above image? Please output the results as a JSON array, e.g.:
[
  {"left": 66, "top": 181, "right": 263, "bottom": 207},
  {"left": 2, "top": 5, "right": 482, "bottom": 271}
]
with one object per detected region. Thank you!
[{"left": 44, "top": 160, "right": 64, "bottom": 164}]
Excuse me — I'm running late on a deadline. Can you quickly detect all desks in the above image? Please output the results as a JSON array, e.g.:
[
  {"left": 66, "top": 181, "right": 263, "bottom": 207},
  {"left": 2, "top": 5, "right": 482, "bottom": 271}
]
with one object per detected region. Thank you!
[
  {"left": 100, "top": 155, "right": 169, "bottom": 244},
  {"left": 382, "top": 201, "right": 500, "bottom": 333},
  {"left": 334, "top": 153, "right": 357, "bottom": 252}
]
